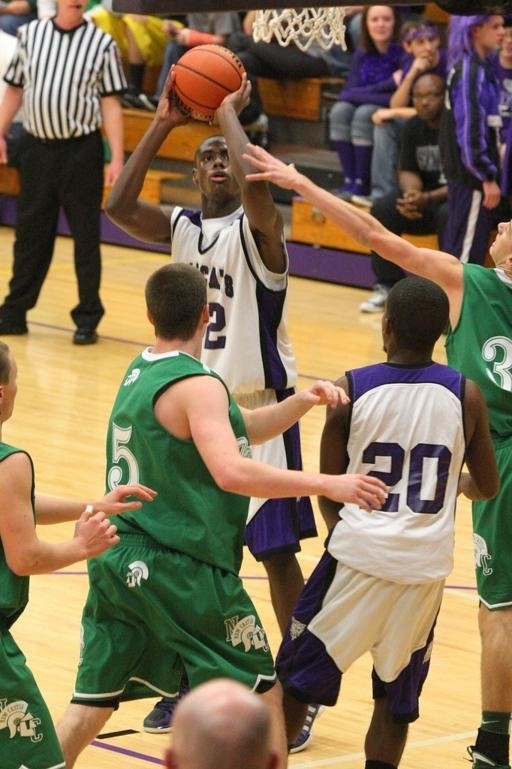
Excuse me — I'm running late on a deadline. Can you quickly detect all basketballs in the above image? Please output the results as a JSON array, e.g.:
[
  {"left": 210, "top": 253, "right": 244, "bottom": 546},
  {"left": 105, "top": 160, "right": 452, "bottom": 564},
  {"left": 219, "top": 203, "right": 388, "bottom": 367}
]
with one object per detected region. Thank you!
[{"left": 169, "top": 43, "right": 249, "bottom": 124}]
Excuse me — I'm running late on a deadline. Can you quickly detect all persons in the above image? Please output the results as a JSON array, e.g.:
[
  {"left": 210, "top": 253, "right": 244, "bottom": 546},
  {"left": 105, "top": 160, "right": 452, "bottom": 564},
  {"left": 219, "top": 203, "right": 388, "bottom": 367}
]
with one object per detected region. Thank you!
[
  {"left": 275, "top": 275, "right": 501, "bottom": 769},
  {"left": 1, "top": 339, "right": 158, "bottom": 769},
  {"left": 104, "top": 64, "right": 325, "bottom": 755},
  {"left": 55, "top": 262, "right": 389, "bottom": 769},
  {"left": 162, "top": 677, "right": 288, "bottom": 769},
  {"left": 242, "top": 143, "right": 512, "bottom": 769},
  {"left": 2, "top": 1, "right": 512, "bottom": 344}
]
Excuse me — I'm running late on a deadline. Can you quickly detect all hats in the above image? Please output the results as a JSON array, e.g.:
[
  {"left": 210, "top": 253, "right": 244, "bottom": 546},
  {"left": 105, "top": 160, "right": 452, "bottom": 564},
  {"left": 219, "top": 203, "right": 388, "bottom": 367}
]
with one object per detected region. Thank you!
[{"left": 404, "top": 26, "right": 439, "bottom": 42}]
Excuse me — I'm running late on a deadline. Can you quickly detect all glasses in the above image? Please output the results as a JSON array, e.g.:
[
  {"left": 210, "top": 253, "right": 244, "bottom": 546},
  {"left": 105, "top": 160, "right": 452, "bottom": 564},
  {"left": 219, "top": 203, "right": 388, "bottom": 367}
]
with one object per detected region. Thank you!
[{"left": 410, "top": 91, "right": 440, "bottom": 101}]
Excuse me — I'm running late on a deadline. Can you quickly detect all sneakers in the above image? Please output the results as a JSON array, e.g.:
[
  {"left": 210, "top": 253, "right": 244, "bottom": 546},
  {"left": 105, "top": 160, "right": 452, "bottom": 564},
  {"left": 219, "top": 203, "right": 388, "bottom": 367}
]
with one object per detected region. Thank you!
[
  {"left": 352, "top": 193, "right": 378, "bottom": 209},
  {"left": 0, "top": 320, "right": 30, "bottom": 335},
  {"left": 289, "top": 699, "right": 326, "bottom": 755},
  {"left": 142, "top": 680, "right": 192, "bottom": 736},
  {"left": 72, "top": 325, "right": 98, "bottom": 345},
  {"left": 358, "top": 284, "right": 392, "bottom": 314}
]
